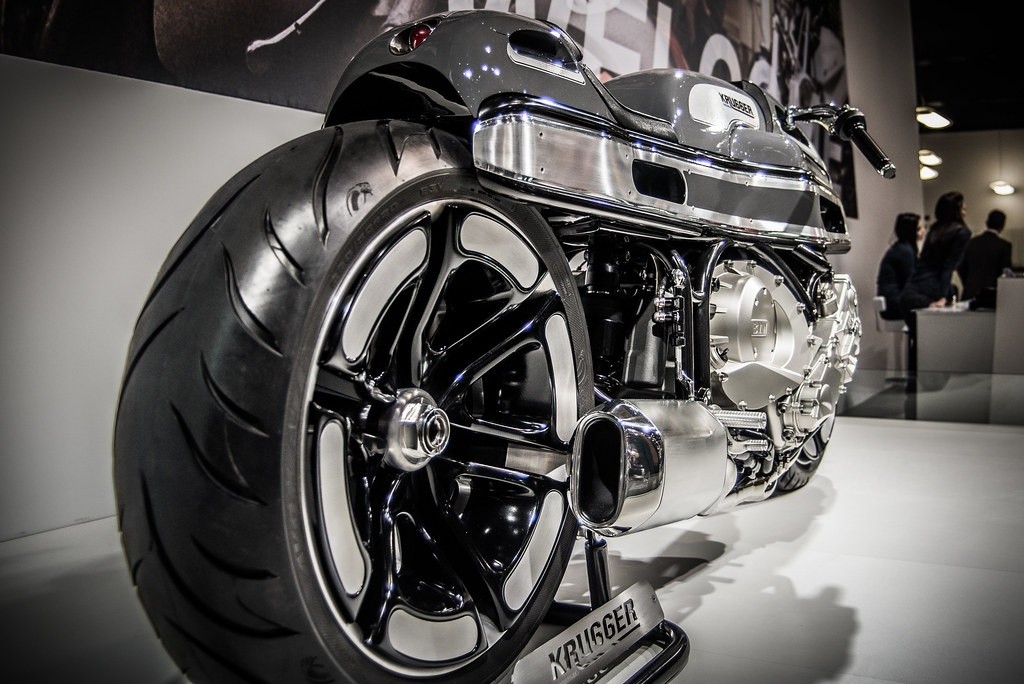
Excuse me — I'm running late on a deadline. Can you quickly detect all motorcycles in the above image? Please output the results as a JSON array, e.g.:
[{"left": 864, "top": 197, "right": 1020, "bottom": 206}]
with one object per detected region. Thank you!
[{"left": 110, "top": 1, "right": 898, "bottom": 683}]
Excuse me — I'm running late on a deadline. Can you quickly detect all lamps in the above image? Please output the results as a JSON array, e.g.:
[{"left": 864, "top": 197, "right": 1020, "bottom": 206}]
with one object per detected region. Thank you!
[
  {"left": 916, "top": 92, "right": 953, "bottom": 128},
  {"left": 989, "top": 132, "right": 1015, "bottom": 196},
  {"left": 918, "top": 149, "right": 943, "bottom": 166}
]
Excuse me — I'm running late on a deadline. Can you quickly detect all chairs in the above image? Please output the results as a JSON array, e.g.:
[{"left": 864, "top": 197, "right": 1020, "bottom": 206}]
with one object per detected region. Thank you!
[{"left": 873, "top": 297, "right": 908, "bottom": 380}]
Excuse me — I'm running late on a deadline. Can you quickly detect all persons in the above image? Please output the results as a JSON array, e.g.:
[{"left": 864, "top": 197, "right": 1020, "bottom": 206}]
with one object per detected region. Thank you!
[
  {"left": 876, "top": 213, "right": 925, "bottom": 396},
  {"left": 962, "top": 210, "right": 1012, "bottom": 311},
  {"left": 913, "top": 191, "right": 972, "bottom": 310}
]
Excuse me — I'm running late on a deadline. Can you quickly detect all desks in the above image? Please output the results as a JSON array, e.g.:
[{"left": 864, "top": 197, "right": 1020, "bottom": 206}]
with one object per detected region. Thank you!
[{"left": 917, "top": 297, "right": 1000, "bottom": 422}]
